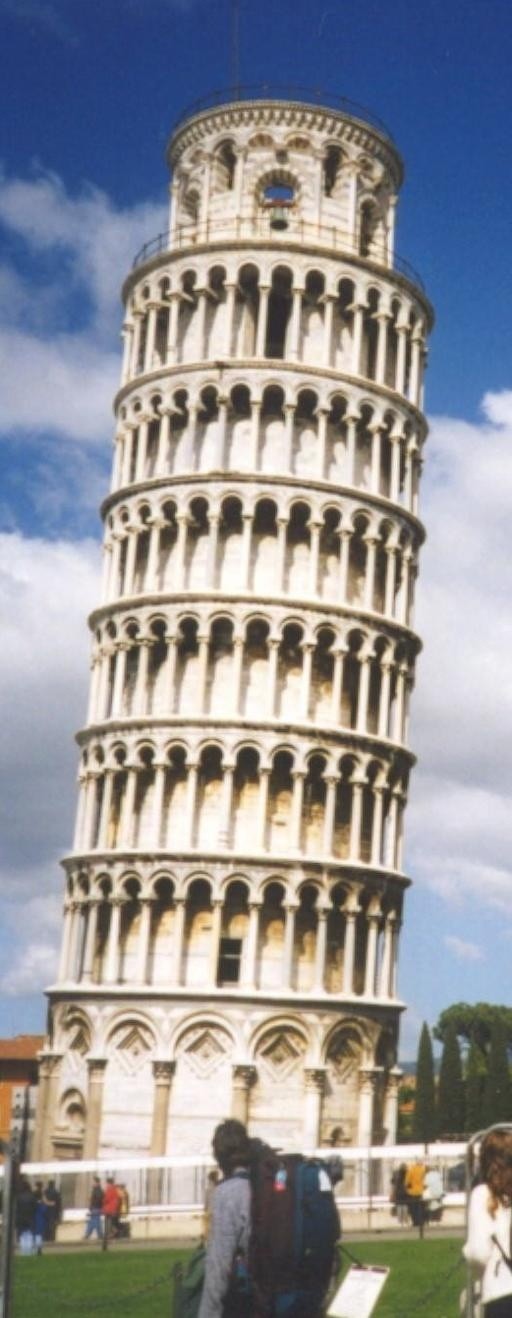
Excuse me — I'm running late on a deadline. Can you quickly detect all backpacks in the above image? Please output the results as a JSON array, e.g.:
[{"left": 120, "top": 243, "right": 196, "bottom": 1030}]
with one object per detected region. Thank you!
[{"left": 241, "top": 1150, "right": 338, "bottom": 1317}]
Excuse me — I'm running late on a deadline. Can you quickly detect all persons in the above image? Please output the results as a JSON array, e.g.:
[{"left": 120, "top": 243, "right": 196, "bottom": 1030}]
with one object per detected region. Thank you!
[
  {"left": 196, "top": 1169, "right": 219, "bottom": 1249},
  {"left": 13, "top": 1173, "right": 129, "bottom": 1255},
  {"left": 458, "top": 1128, "right": 512, "bottom": 1317},
  {"left": 194, "top": 1117, "right": 252, "bottom": 1318},
  {"left": 422, "top": 1168, "right": 445, "bottom": 1224},
  {"left": 390, "top": 1162, "right": 408, "bottom": 1225},
  {"left": 402, "top": 1164, "right": 427, "bottom": 1227}
]
